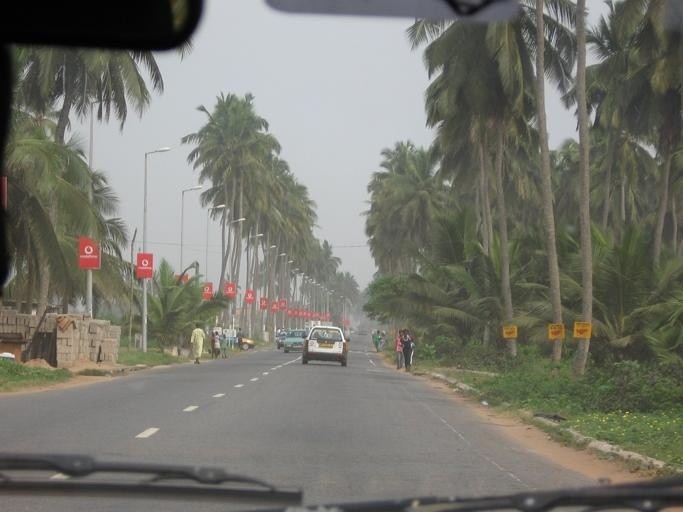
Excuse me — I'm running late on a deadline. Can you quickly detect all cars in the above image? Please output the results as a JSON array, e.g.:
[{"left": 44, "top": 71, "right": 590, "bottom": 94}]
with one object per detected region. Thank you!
[
  {"left": 281, "top": 328, "right": 307, "bottom": 354},
  {"left": 240, "top": 336, "right": 256, "bottom": 351},
  {"left": 275, "top": 330, "right": 286, "bottom": 350}
]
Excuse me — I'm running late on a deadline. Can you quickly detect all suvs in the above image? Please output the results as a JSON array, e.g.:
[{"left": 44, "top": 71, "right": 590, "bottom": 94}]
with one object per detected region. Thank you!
[{"left": 302, "top": 325, "right": 350, "bottom": 367}]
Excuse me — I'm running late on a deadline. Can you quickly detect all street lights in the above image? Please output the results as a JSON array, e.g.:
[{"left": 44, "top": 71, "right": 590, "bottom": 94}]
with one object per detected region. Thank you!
[
  {"left": 229, "top": 215, "right": 353, "bottom": 342},
  {"left": 142, "top": 147, "right": 170, "bottom": 355},
  {"left": 85, "top": 98, "right": 111, "bottom": 323},
  {"left": 180, "top": 185, "right": 202, "bottom": 280},
  {"left": 204, "top": 204, "right": 225, "bottom": 286}
]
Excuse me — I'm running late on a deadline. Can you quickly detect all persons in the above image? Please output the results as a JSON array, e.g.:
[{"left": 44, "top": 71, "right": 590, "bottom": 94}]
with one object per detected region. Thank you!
[
  {"left": 190, "top": 323, "right": 243, "bottom": 364},
  {"left": 372, "top": 329, "right": 415, "bottom": 372}
]
